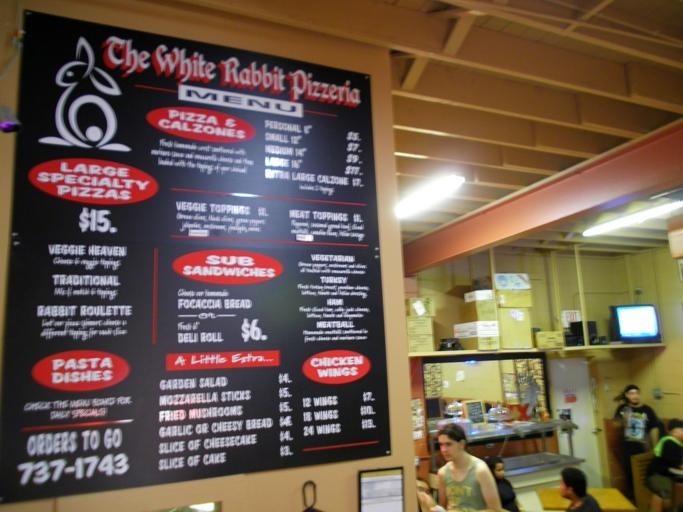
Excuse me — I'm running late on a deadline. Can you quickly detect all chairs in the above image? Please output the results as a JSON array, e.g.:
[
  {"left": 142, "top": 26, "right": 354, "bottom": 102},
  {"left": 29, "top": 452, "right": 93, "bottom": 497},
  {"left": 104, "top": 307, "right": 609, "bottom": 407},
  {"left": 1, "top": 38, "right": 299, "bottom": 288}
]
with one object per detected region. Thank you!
[{"left": 632, "top": 450, "right": 674, "bottom": 511}]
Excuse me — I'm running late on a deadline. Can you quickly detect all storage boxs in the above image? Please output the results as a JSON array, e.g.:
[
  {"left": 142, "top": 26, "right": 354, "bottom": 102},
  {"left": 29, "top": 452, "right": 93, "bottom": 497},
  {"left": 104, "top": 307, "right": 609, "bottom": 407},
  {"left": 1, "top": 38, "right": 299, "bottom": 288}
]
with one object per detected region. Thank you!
[
  {"left": 454, "top": 272, "right": 534, "bottom": 349},
  {"left": 534, "top": 331, "right": 566, "bottom": 349},
  {"left": 405, "top": 297, "right": 437, "bottom": 352}
]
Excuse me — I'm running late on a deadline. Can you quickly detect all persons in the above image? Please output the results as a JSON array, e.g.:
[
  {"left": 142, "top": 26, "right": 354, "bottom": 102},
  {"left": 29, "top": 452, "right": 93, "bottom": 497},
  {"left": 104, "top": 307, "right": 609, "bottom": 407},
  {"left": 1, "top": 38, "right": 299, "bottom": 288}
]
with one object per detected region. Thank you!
[
  {"left": 483, "top": 455, "right": 520, "bottom": 512},
  {"left": 610, "top": 382, "right": 659, "bottom": 502},
  {"left": 558, "top": 466, "right": 602, "bottom": 512},
  {"left": 434, "top": 423, "right": 504, "bottom": 512},
  {"left": 644, "top": 419, "right": 682, "bottom": 512}
]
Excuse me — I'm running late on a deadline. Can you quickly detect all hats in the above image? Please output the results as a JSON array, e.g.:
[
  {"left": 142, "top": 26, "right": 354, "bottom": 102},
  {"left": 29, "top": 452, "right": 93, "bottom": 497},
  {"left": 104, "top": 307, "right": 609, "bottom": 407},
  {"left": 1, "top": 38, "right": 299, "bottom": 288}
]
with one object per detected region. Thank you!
[{"left": 667, "top": 418, "right": 683, "bottom": 429}]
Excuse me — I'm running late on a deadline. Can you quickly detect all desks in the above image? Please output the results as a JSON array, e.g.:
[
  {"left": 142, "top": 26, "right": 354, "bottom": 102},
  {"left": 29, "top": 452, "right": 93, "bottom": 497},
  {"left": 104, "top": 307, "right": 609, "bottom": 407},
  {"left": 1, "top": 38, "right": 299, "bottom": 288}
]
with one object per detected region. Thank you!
[{"left": 535, "top": 484, "right": 640, "bottom": 511}]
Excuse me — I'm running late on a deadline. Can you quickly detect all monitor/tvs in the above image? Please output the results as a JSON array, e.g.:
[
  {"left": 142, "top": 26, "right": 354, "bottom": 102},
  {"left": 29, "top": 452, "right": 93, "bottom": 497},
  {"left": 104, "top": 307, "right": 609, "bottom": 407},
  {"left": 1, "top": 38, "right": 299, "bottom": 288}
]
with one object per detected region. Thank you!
[{"left": 613, "top": 304, "right": 661, "bottom": 344}]
